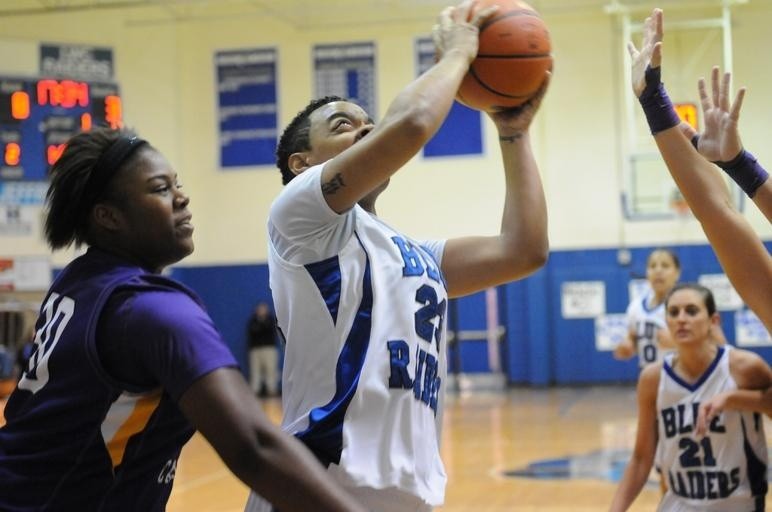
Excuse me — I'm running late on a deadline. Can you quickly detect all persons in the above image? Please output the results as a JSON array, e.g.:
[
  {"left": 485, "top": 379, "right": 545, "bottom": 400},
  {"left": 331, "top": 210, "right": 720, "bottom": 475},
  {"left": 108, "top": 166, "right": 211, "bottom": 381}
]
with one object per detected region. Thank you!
[
  {"left": 598, "top": 283, "right": 772, "bottom": 511},
  {"left": 1, "top": 126, "right": 369, "bottom": 512},
  {"left": 694, "top": 380, "right": 771, "bottom": 443},
  {"left": 611, "top": 243, "right": 730, "bottom": 364},
  {"left": 242, "top": 300, "right": 283, "bottom": 394},
  {"left": 624, "top": 6, "right": 771, "bottom": 335},
  {"left": 240, "top": 0, "right": 553, "bottom": 511},
  {"left": 15, "top": 325, "right": 38, "bottom": 369}
]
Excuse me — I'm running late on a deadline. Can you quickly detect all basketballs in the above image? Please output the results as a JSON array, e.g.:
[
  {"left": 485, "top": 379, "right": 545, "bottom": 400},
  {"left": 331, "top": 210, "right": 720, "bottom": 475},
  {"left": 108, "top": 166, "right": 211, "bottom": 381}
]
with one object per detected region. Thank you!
[{"left": 435, "top": 1, "right": 551, "bottom": 112}]
